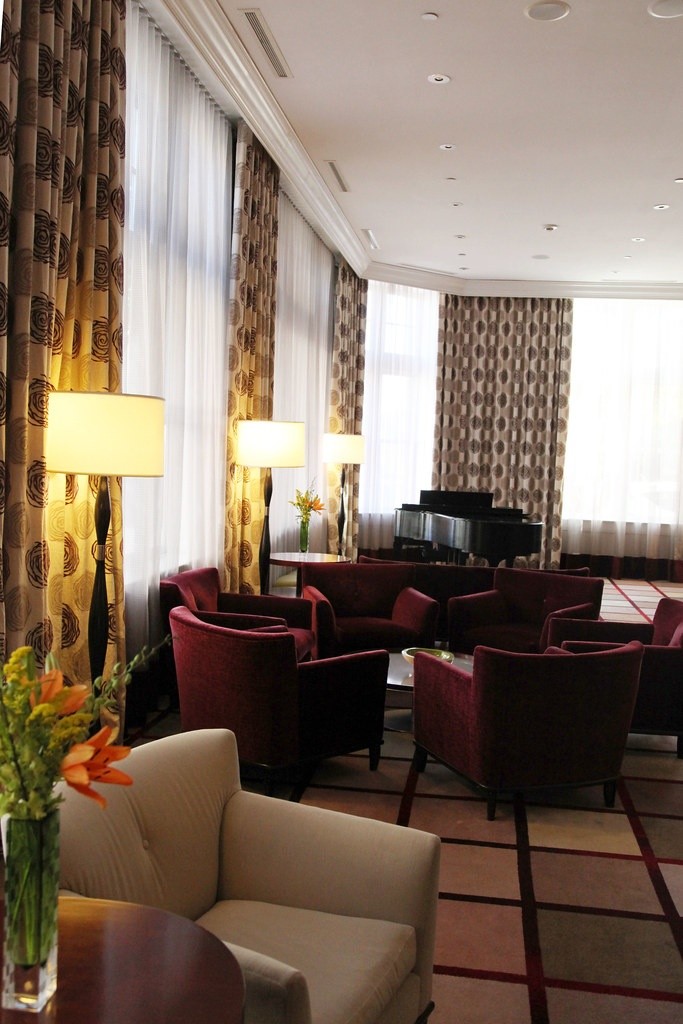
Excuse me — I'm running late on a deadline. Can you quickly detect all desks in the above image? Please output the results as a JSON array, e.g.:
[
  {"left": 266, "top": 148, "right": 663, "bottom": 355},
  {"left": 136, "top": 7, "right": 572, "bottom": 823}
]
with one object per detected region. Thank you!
[{"left": 270, "top": 552, "right": 351, "bottom": 597}]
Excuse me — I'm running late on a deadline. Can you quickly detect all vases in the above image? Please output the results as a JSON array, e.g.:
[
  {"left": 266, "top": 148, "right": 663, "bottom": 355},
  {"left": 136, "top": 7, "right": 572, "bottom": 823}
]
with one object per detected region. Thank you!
[
  {"left": 1, "top": 808, "right": 80, "bottom": 1012},
  {"left": 299, "top": 518, "right": 309, "bottom": 553}
]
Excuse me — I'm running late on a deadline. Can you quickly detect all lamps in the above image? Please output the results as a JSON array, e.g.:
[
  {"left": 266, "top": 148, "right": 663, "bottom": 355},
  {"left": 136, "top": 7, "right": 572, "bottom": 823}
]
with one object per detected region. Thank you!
[
  {"left": 232, "top": 420, "right": 312, "bottom": 592},
  {"left": 42, "top": 392, "right": 167, "bottom": 738},
  {"left": 323, "top": 432, "right": 370, "bottom": 555}
]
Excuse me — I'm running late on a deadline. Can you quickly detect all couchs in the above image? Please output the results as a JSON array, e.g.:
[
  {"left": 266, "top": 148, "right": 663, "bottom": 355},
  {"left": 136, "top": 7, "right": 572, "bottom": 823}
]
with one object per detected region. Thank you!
[{"left": 56, "top": 562, "right": 682, "bottom": 1024}]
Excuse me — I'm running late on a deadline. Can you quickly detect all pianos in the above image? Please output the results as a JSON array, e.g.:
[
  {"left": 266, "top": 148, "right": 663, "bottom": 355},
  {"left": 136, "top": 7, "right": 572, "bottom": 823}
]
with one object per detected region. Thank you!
[{"left": 393, "top": 490, "right": 544, "bottom": 569}]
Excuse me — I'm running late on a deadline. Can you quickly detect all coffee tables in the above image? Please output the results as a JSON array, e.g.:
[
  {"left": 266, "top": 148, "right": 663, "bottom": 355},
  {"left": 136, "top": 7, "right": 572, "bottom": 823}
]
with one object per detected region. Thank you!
[{"left": 387, "top": 648, "right": 476, "bottom": 690}]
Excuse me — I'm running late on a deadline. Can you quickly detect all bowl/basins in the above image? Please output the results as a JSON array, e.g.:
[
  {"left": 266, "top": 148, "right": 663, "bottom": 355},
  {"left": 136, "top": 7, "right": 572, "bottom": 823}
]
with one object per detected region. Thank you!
[{"left": 402, "top": 647, "right": 455, "bottom": 665}]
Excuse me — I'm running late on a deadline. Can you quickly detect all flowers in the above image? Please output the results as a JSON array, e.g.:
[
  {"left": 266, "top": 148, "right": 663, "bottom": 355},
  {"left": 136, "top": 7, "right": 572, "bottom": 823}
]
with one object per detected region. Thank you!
[
  {"left": 0, "top": 635, "right": 163, "bottom": 832},
  {"left": 289, "top": 476, "right": 326, "bottom": 520}
]
